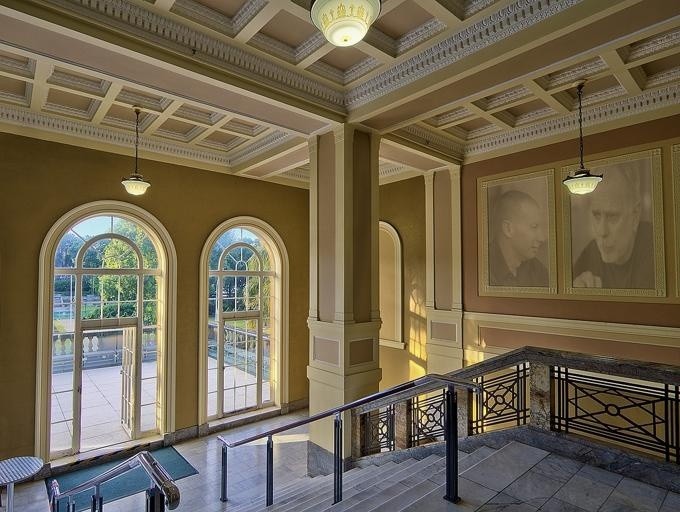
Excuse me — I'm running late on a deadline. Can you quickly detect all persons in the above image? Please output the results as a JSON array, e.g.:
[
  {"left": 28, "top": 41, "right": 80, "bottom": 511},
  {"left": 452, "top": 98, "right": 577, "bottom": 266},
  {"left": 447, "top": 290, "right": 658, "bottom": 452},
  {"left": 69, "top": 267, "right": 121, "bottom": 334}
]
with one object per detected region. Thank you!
[
  {"left": 489, "top": 189, "right": 548, "bottom": 287},
  {"left": 571, "top": 160, "right": 657, "bottom": 290}
]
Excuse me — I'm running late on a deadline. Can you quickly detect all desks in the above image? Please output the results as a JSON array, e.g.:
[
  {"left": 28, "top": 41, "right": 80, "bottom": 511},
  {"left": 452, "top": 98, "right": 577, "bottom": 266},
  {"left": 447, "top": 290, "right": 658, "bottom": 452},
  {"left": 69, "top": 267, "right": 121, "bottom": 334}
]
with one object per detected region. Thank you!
[{"left": 0, "top": 455, "right": 44, "bottom": 512}]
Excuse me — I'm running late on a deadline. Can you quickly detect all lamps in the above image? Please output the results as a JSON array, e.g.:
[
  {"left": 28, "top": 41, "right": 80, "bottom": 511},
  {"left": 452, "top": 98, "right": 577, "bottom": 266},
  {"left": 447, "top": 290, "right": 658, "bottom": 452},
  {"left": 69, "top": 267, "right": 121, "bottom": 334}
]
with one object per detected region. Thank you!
[
  {"left": 309, "top": 0, "right": 381, "bottom": 47},
  {"left": 562, "top": 83, "right": 602, "bottom": 196},
  {"left": 119, "top": 106, "right": 152, "bottom": 197}
]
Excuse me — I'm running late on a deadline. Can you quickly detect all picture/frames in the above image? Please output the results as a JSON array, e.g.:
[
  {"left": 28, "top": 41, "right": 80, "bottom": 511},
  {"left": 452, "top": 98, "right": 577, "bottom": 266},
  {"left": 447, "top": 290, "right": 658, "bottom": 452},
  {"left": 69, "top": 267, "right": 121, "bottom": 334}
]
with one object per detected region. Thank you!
[
  {"left": 478, "top": 169, "right": 558, "bottom": 295},
  {"left": 562, "top": 148, "right": 667, "bottom": 298}
]
